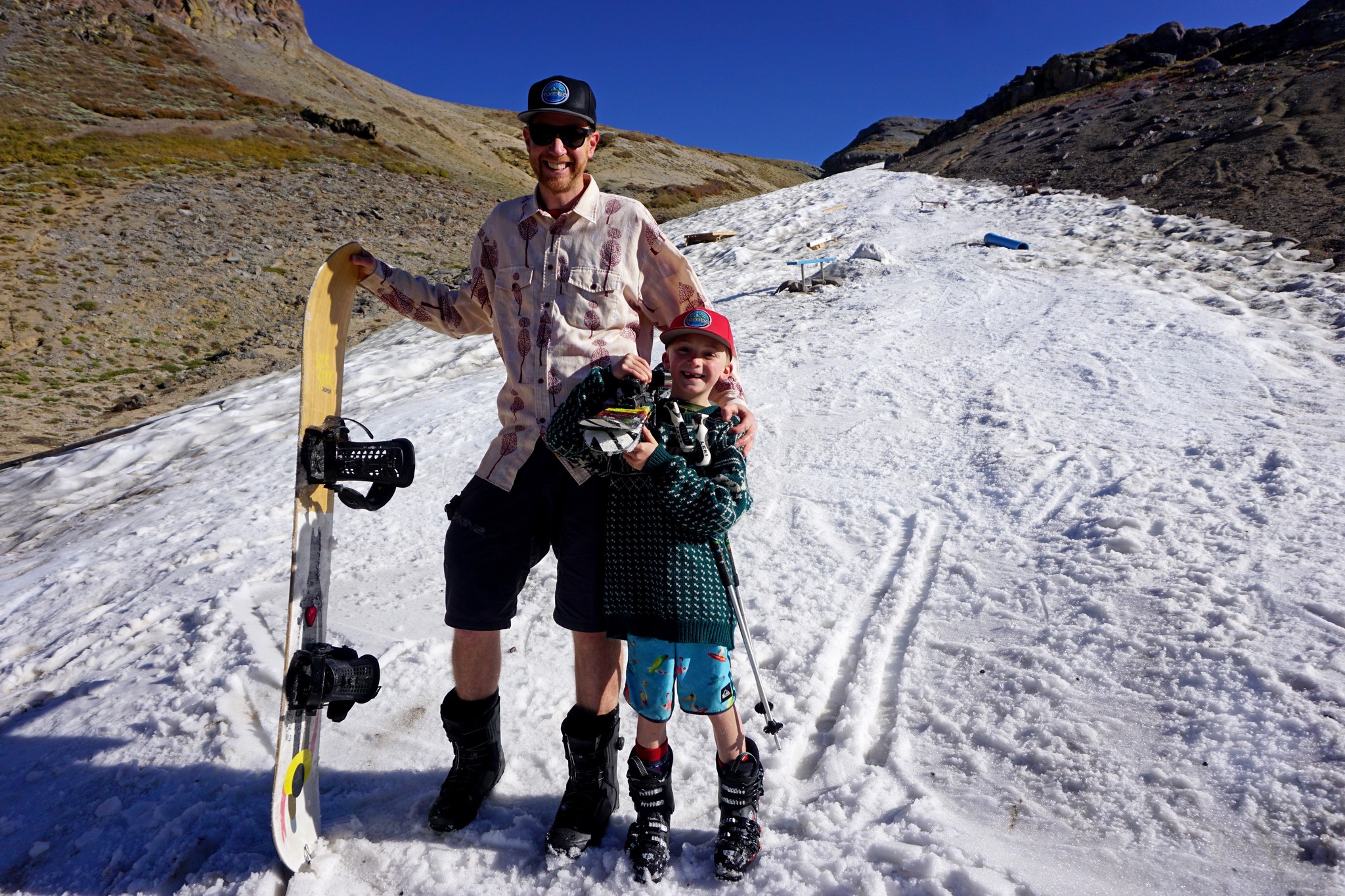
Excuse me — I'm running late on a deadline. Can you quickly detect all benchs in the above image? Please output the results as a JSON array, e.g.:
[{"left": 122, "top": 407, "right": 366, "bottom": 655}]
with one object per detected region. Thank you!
[
  {"left": 920, "top": 201, "right": 948, "bottom": 210},
  {"left": 787, "top": 258, "right": 835, "bottom": 292}
]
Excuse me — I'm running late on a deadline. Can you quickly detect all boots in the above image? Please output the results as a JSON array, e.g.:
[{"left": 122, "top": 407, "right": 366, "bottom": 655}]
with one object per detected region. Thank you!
[
  {"left": 626, "top": 744, "right": 675, "bottom": 884},
  {"left": 545, "top": 703, "right": 625, "bottom": 861},
  {"left": 714, "top": 736, "right": 764, "bottom": 881},
  {"left": 428, "top": 685, "right": 506, "bottom": 832}
]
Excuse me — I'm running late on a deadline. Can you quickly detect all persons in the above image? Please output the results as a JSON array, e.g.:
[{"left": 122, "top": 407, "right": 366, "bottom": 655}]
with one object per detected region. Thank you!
[
  {"left": 543, "top": 308, "right": 766, "bottom": 884},
  {"left": 350, "top": 75, "right": 757, "bottom": 860}
]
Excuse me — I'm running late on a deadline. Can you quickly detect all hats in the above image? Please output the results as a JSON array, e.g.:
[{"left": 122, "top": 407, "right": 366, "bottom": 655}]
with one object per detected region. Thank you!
[
  {"left": 660, "top": 308, "right": 733, "bottom": 363},
  {"left": 518, "top": 75, "right": 597, "bottom": 132}
]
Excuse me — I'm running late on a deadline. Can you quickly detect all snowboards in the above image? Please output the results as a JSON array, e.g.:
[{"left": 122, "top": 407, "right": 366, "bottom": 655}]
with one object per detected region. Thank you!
[{"left": 270, "top": 241, "right": 362, "bottom": 875}]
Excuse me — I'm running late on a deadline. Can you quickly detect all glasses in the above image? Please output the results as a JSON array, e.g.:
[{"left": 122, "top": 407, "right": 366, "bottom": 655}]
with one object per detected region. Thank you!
[{"left": 527, "top": 123, "right": 594, "bottom": 148}]
